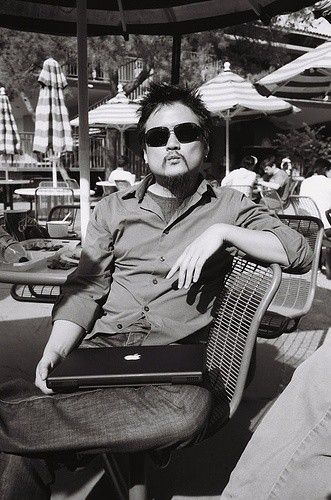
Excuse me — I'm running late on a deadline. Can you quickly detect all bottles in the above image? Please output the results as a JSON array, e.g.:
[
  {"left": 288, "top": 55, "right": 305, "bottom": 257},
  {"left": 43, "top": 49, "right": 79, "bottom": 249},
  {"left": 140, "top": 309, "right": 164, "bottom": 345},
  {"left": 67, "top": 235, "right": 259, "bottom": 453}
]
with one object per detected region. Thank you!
[{"left": 0, "top": 225, "right": 28, "bottom": 264}]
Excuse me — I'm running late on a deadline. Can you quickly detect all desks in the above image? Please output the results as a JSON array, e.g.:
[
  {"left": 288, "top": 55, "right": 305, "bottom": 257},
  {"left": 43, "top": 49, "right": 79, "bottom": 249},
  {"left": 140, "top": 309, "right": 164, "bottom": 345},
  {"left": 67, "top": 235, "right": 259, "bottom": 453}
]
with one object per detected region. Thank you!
[
  {"left": 0, "top": 238, "right": 82, "bottom": 286},
  {"left": 0, "top": 180, "right": 30, "bottom": 226},
  {"left": 252, "top": 185, "right": 278, "bottom": 194},
  {"left": 14, "top": 189, "right": 94, "bottom": 220},
  {"left": 96, "top": 181, "right": 116, "bottom": 186}
]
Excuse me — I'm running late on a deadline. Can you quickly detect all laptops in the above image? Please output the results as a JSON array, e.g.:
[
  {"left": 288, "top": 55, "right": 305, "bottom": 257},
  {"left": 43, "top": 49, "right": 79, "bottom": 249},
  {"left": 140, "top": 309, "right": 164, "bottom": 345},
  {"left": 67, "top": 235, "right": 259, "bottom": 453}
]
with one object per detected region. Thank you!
[{"left": 46, "top": 344, "right": 205, "bottom": 392}]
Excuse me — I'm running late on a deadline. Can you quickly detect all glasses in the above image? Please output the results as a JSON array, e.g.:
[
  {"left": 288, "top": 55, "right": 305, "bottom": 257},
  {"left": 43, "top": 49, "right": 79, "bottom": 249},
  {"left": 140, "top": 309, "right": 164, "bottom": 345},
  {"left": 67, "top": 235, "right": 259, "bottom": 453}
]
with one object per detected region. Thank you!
[{"left": 143, "top": 122, "right": 209, "bottom": 147}]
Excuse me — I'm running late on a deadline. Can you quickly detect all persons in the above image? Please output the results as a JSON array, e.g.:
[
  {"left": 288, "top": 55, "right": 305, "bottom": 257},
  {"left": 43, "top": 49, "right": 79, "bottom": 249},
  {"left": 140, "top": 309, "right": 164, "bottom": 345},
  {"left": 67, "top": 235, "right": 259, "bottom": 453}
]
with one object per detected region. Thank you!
[
  {"left": 299, "top": 158, "right": 331, "bottom": 278},
  {"left": 221, "top": 155, "right": 291, "bottom": 212},
  {"left": 109, "top": 155, "right": 132, "bottom": 183},
  {"left": 220, "top": 342, "right": 331, "bottom": 500},
  {"left": 0, "top": 83, "right": 314, "bottom": 500}
]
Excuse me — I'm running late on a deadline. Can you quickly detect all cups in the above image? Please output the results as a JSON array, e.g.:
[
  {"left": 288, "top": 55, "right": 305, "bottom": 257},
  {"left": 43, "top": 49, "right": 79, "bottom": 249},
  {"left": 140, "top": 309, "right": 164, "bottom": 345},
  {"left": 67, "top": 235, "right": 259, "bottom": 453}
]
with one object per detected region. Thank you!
[{"left": 4, "top": 210, "right": 28, "bottom": 240}]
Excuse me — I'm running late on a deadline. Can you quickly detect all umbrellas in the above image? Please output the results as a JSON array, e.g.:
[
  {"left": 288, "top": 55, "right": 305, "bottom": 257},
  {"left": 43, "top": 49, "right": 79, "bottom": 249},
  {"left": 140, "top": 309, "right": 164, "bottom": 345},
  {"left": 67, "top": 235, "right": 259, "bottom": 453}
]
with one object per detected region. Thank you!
[
  {"left": 32, "top": 57, "right": 72, "bottom": 218},
  {"left": 0, "top": 0, "right": 323, "bottom": 248},
  {"left": 0, "top": 83, "right": 22, "bottom": 203},
  {"left": 195, "top": 62, "right": 302, "bottom": 175},
  {"left": 254, "top": 42, "right": 331, "bottom": 101},
  {"left": 70, "top": 84, "right": 142, "bottom": 155}
]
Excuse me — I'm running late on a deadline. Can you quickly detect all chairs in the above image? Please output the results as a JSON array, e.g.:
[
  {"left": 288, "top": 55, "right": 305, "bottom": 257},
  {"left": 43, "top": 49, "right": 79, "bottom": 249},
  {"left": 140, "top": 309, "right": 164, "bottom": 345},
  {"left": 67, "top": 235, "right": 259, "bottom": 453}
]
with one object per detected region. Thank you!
[{"left": 0, "top": 178, "right": 331, "bottom": 500}]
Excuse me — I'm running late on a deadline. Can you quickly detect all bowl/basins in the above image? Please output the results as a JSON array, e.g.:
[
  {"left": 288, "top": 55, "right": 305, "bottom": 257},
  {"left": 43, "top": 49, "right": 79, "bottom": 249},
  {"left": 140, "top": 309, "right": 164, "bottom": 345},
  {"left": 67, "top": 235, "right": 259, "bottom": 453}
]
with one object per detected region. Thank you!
[{"left": 47, "top": 220, "right": 68, "bottom": 238}]
